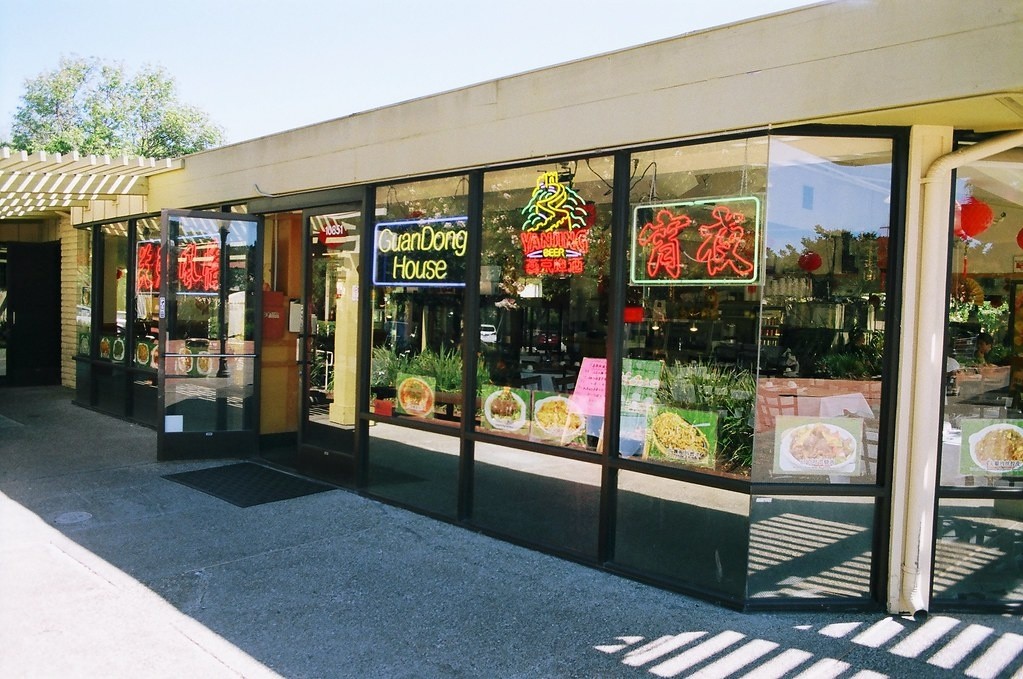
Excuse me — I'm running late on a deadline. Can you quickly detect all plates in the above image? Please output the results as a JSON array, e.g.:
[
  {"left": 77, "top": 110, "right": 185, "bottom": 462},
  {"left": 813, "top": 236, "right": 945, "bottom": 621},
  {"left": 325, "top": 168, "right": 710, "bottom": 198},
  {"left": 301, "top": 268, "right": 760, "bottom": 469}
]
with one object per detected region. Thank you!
[
  {"left": 196, "top": 351, "right": 213, "bottom": 375},
  {"left": 783, "top": 422, "right": 857, "bottom": 472},
  {"left": 136, "top": 342, "right": 150, "bottom": 364},
  {"left": 178, "top": 348, "right": 193, "bottom": 372},
  {"left": 112, "top": 339, "right": 125, "bottom": 360},
  {"left": 100, "top": 337, "right": 110, "bottom": 358},
  {"left": 398, "top": 378, "right": 434, "bottom": 417},
  {"left": 484, "top": 390, "right": 526, "bottom": 431},
  {"left": 150, "top": 345, "right": 158, "bottom": 369},
  {"left": 533, "top": 396, "right": 586, "bottom": 436},
  {"left": 651, "top": 413, "right": 710, "bottom": 464},
  {"left": 969, "top": 424, "right": 1023, "bottom": 472}
]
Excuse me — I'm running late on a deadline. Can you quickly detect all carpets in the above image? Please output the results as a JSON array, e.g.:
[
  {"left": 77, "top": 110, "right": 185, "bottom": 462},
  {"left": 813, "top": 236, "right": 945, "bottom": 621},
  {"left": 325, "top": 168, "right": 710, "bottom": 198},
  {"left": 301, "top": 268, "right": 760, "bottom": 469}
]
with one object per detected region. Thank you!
[{"left": 160, "top": 462, "right": 338, "bottom": 508}]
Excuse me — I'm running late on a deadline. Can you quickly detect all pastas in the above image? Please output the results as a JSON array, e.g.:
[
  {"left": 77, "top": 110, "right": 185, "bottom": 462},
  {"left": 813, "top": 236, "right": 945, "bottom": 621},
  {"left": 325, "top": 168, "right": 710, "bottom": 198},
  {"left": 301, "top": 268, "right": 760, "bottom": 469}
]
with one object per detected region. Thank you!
[{"left": 653, "top": 412, "right": 708, "bottom": 457}]
